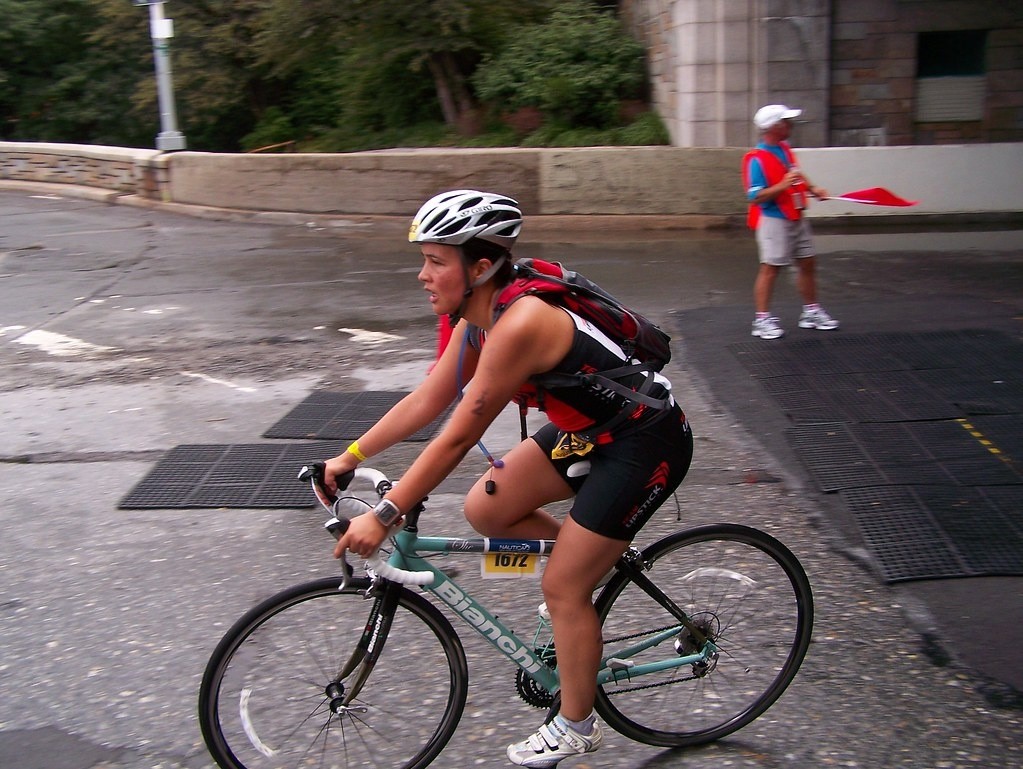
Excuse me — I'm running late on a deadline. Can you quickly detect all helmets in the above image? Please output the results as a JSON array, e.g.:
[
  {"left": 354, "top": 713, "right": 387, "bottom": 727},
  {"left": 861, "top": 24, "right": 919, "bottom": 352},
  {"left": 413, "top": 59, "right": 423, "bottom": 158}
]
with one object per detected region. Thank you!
[{"left": 409, "top": 188, "right": 522, "bottom": 250}]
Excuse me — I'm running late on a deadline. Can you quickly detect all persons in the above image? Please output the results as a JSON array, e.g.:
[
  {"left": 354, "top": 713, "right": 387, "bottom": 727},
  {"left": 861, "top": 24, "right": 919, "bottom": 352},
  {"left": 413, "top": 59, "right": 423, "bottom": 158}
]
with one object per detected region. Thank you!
[
  {"left": 313, "top": 189, "right": 694, "bottom": 769},
  {"left": 742, "top": 104, "right": 839, "bottom": 338}
]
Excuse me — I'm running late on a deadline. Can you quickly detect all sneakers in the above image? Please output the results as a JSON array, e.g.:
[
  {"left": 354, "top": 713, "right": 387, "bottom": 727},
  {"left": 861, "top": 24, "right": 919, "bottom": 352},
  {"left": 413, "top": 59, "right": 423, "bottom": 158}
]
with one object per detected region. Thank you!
[
  {"left": 751, "top": 316, "right": 785, "bottom": 339},
  {"left": 798, "top": 308, "right": 839, "bottom": 330},
  {"left": 537, "top": 566, "right": 617, "bottom": 620},
  {"left": 505, "top": 716, "right": 602, "bottom": 768}
]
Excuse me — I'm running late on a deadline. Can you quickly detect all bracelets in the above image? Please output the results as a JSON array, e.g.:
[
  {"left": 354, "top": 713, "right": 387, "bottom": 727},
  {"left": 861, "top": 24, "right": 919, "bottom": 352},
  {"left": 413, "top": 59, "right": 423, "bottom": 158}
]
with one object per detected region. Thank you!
[
  {"left": 348, "top": 442, "right": 368, "bottom": 462},
  {"left": 808, "top": 185, "right": 816, "bottom": 192}
]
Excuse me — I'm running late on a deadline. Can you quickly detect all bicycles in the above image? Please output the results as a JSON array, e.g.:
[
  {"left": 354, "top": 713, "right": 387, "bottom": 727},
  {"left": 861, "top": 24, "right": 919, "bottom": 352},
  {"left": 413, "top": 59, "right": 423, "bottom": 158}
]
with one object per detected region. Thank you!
[{"left": 198, "top": 460, "right": 813, "bottom": 768}]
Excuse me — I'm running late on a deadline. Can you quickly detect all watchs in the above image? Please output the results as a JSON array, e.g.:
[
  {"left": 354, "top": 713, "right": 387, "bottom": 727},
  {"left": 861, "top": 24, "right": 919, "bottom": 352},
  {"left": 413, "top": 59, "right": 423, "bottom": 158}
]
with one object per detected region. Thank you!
[{"left": 373, "top": 498, "right": 405, "bottom": 527}]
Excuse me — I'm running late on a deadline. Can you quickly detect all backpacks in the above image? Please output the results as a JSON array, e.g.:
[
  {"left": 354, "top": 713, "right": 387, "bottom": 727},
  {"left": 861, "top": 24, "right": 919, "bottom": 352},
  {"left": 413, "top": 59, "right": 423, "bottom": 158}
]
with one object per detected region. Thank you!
[{"left": 467, "top": 258, "right": 671, "bottom": 388}]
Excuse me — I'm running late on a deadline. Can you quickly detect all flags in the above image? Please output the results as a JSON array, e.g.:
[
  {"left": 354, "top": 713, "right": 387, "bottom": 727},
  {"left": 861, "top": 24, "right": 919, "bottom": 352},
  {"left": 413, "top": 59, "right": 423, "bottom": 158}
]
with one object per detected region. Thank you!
[{"left": 840, "top": 188, "right": 918, "bottom": 208}]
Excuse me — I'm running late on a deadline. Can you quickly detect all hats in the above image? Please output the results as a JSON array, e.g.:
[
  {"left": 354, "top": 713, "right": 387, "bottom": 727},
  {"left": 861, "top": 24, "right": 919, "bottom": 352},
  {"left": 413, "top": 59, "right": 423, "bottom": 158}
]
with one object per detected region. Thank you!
[{"left": 753, "top": 105, "right": 801, "bottom": 130}]
